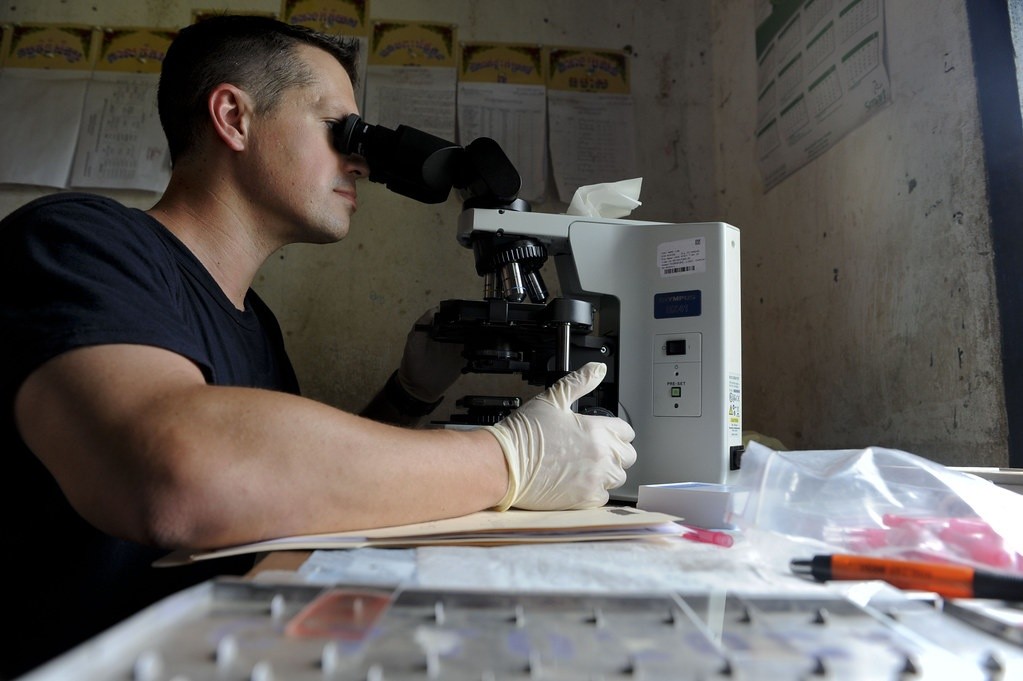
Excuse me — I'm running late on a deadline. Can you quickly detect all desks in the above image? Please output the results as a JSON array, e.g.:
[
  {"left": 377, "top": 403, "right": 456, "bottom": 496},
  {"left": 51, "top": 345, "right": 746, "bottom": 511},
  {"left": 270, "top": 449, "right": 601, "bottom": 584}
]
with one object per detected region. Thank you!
[{"left": 242, "top": 512, "right": 1023, "bottom": 625}]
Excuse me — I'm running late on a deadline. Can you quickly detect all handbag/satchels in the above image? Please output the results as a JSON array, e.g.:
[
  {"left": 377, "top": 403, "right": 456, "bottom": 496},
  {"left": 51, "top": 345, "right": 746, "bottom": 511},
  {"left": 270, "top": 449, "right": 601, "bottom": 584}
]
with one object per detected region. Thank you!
[{"left": 731, "top": 440, "right": 1023, "bottom": 583}]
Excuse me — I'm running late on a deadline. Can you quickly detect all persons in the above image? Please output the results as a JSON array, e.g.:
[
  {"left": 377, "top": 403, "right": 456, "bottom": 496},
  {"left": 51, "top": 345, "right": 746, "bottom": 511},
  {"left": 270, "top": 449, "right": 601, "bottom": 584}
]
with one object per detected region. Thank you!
[{"left": 0, "top": 14, "right": 637, "bottom": 681}]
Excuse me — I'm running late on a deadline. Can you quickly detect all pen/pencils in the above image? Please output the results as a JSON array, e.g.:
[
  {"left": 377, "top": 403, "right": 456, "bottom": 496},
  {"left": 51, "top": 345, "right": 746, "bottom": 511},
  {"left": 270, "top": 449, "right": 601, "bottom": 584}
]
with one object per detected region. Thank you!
[
  {"left": 652, "top": 521, "right": 734, "bottom": 549},
  {"left": 789, "top": 555, "right": 1023, "bottom": 601}
]
